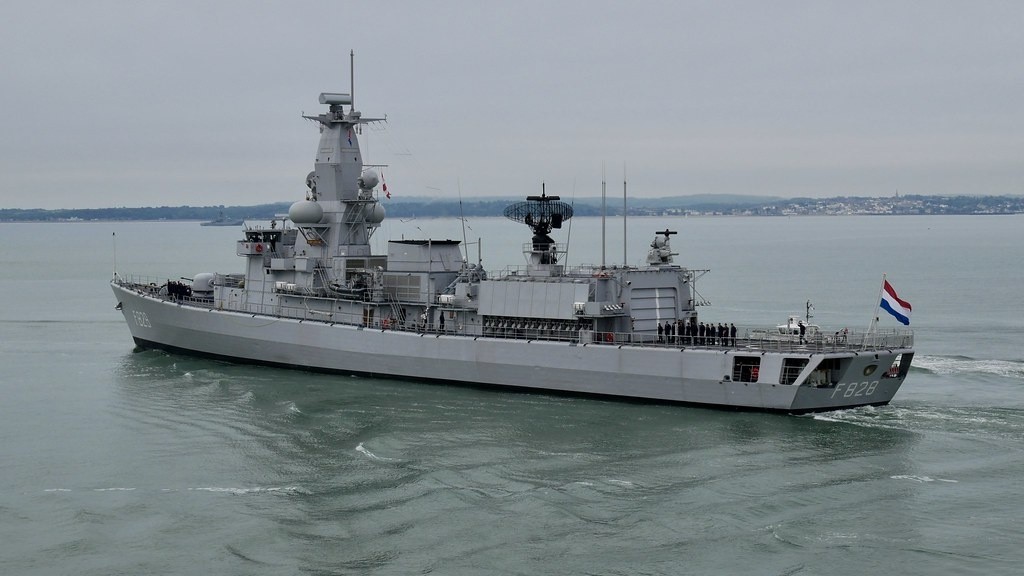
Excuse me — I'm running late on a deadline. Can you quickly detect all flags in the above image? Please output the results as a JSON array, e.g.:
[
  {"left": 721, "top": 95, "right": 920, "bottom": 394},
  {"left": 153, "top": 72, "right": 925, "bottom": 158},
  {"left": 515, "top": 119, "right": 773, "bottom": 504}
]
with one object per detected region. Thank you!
[{"left": 879, "top": 273, "right": 913, "bottom": 326}]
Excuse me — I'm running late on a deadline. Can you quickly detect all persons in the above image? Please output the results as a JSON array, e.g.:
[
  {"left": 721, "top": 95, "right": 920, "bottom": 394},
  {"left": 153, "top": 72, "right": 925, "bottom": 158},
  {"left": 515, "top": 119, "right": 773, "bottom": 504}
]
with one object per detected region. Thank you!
[
  {"left": 167, "top": 279, "right": 191, "bottom": 300},
  {"left": 658, "top": 321, "right": 737, "bottom": 347},
  {"left": 439, "top": 312, "right": 445, "bottom": 334},
  {"left": 798, "top": 323, "right": 808, "bottom": 345},
  {"left": 841, "top": 327, "right": 848, "bottom": 343}
]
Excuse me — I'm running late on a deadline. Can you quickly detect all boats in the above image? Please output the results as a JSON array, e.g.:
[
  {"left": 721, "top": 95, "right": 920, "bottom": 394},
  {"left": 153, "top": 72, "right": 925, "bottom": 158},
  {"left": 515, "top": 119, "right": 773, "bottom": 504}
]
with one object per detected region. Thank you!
[
  {"left": 108, "top": 48, "right": 915, "bottom": 416},
  {"left": 200, "top": 208, "right": 245, "bottom": 226}
]
